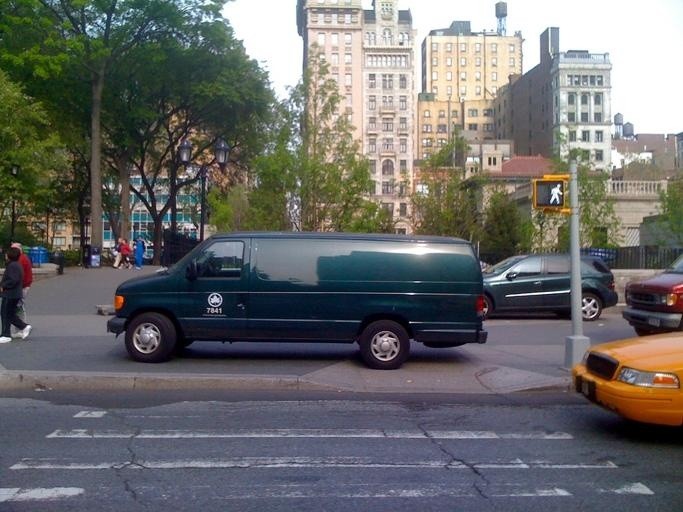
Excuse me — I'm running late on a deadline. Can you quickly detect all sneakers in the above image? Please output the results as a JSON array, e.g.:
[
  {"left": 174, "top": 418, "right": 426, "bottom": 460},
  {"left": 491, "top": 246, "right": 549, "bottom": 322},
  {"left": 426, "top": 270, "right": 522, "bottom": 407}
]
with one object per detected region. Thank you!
[{"left": 0, "top": 325, "right": 32, "bottom": 343}]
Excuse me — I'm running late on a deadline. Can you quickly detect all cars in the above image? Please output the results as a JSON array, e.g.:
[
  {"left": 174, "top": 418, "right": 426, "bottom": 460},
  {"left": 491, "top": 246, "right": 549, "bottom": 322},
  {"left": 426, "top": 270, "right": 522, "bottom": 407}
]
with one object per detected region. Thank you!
[{"left": 564, "top": 331, "right": 682, "bottom": 431}]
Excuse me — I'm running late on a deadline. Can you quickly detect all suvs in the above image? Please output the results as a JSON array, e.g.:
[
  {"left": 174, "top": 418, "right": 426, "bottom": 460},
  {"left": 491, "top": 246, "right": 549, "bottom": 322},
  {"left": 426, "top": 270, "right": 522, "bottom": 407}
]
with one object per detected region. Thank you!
[{"left": 624, "top": 252, "right": 682, "bottom": 336}]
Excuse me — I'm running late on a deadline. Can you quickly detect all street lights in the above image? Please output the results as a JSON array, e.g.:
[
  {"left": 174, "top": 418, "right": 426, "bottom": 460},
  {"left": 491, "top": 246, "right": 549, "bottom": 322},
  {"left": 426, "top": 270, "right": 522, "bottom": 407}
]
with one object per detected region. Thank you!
[
  {"left": 176, "top": 138, "right": 230, "bottom": 237},
  {"left": 530, "top": 176, "right": 574, "bottom": 215},
  {"left": 607, "top": 114, "right": 636, "bottom": 182},
  {"left": 7, "top": 159, "right": 19, "bottom": 266}
]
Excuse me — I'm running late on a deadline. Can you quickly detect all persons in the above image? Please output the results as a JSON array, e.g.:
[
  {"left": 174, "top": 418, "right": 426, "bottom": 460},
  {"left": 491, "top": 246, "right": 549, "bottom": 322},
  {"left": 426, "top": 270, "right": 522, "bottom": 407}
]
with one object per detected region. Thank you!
[
  {"left": 131, "top": 235, "right": 146, "bottom": 270},
  {"left": 111, "top": 237, "right": 128, "bottom": 269},
  {"left": 0, "top": 247, "right": 32, "bottom": 343},
  {"left": 9, "top": 242, "right": 33, "bottom": 338},
  {"left": 115, "top": 238, "right": 132, "bottom": 270}
]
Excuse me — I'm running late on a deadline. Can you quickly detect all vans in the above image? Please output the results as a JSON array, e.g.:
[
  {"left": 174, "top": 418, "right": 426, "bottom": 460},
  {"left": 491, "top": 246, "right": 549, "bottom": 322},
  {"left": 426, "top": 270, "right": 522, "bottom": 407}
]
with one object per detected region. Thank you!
[
  {"left": 479, "top": 250, "right": 619, "bottom": 324},
  {"left": 102, "top": 232, "right": 489, "bottom": 372}
]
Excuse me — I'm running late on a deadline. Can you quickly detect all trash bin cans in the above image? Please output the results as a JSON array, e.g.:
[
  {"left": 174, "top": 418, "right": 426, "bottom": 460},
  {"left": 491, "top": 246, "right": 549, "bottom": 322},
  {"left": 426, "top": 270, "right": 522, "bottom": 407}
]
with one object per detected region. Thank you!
[{"left": 49, "top": 256, "right": 66, "bottom": 275}]
